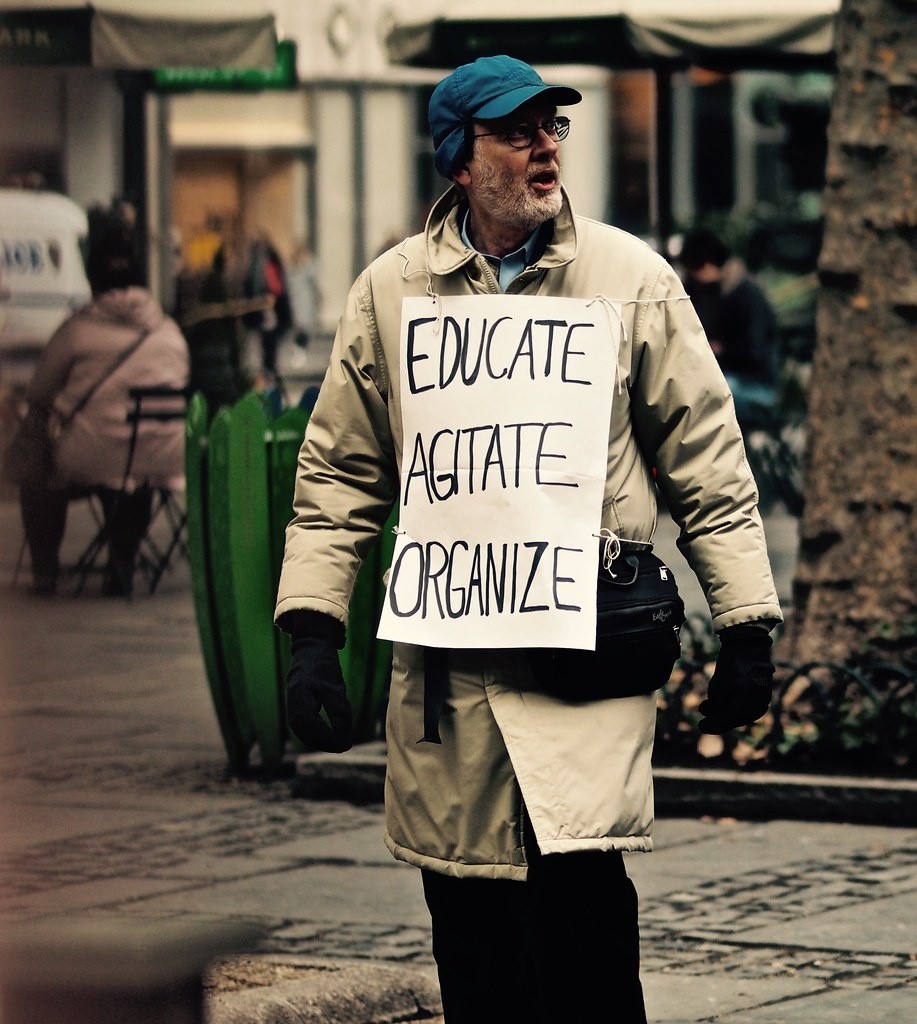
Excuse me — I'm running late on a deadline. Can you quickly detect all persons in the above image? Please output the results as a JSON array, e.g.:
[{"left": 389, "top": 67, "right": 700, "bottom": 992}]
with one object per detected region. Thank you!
[
  {"left": 0, "top": 167, "right": 291, "bottom": 592},
  {"left": 273, "top": 53, "right": 786, "bottom": 1024},
  {"left": 678, "top": 243, "right": 780, "bottom": 407}
]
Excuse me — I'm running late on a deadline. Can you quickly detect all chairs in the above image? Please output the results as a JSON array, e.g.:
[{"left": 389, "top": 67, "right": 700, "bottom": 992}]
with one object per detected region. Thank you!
[{"left": 8, "top": 380, "right": 193, "bottom": 601}]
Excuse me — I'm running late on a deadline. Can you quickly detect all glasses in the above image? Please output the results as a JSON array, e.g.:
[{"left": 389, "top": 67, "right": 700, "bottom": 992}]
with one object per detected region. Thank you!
[{"left": 474, "top": 116, "right": 571, "bottom": 149}]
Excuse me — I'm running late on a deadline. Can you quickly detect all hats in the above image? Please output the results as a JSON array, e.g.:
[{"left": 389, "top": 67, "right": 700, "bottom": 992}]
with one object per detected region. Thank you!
[{"left": 427, "top": 54, "right": 583, "bottom": 178}]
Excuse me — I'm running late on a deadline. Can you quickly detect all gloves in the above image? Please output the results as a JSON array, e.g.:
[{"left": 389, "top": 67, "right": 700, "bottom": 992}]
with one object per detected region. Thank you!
[
  {"left": 286, "top": 620, "right": 357, "bottom": 753},
  {"left": 697, "top": 625, "right": 776, "bottom": 735}
]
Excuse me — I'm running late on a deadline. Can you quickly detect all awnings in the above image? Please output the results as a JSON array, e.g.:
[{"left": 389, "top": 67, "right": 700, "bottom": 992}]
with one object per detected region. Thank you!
[
  {"left": 70, "top": 7, "right": 275, "bottom": 69},
  {"left": 393, "top": 15, "right": 824, "bottom": 65}
]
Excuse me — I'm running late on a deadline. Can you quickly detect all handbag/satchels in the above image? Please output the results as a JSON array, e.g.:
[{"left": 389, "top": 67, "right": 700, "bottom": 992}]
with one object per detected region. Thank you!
[{"left": 547, "top": 543, "right": 686, "bottom": 697}]
